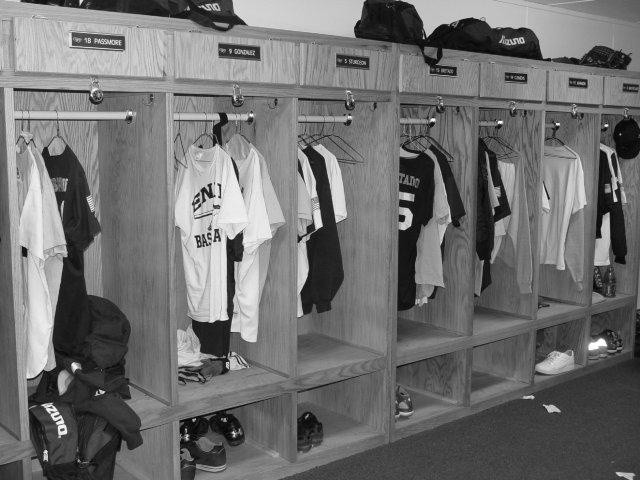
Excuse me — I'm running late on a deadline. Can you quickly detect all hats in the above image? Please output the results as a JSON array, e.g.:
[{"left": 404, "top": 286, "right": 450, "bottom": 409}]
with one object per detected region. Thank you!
[{"left": 612, "top": 118, "right": 640, "bottom": 160}]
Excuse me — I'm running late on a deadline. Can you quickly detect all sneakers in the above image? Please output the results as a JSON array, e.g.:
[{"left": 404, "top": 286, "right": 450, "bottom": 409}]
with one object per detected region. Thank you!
[
  {"left": 597, "top": 330, "right": 618, "bottom": 354},
  {"left": 609, "top": 328, "right": 624, "bottom": 352},
  {"left": 299, "top": 411, "right": 323, "bottom": 448},
  {"left": 596, "top": 333, "right": 608, "bottom": 359},
  {"left": 535, "top": 349, "right": 575, "bottom": 376},
  {"left": 587, "top": 335, "right": 599, "bottom": 361},
  {"left": 394, "top": 392, "right": 400, "bottom": 419},
  {"left": 397, "top": 383, "right": 414, "bottom": 417},
  {"left": 179, "top": 415, "right": 214, "bottom": 442},
  {"left": 180, "top": 435, "right": 227, "bottom": 472},
  {"left": 297, "top": 418, "right": 312, "bottom": 455},
  {"left": 209, "top": 409, "right": 245, "bottom": 447},
  {"left": 180, "top": 446, "right": 196, "bottom": 480}
]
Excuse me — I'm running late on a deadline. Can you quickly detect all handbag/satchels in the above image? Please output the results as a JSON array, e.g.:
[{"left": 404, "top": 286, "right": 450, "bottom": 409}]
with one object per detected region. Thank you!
[
  {"left": 182, "top": 0, "right": 248, "bottom": 32},
  {"left": 490, "top": 26, "right": 543, "bottom": 61},
  {"left": 423, "top": 17, "right": 506, "bottom": 55},
  {"left": 353, "top": 0, "right": 427, "bottom": 46},
  {"left": 577, "top": 46, "right": 632, "bottom": 71}
]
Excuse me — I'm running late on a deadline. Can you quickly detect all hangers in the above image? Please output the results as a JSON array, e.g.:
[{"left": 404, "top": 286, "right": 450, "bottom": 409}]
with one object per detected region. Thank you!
[
  {"left": 546, "top": 121, "right": 579, "bottom": 160},
  {"left": 400, "top": 115, "right": 455, "bottom": 163},
  {"left": 298, "top": 114, "right": 366, "bottom": 164},
  {"left": 15, "top": 109, "right": 69, "bottom": 156},
  {"left": 479, "top": 119, "right": 522, "bottom": 161},
  {"left": 174, "top": 110, "right": 252, "bottom": 172}
]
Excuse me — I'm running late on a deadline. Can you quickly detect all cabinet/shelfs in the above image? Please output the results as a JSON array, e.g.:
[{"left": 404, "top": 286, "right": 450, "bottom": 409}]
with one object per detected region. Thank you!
[
  {"left": 6, "top": 3, "right": 178, "bottom": 480},
  {"left": 466, "top": 53, "right": 545, "bottom": 421},
  {"left": 0, "top": 1, "right": 28, "bottom": 480},
  {"left": 296, "top": 32, "right": 392, "bottom": 472},
  {"left": 582, "top": 68, "right": 640, "bottom": 373},
  {"left": 170, "top": 15, "right": 295, "bottom": 479},
  {"left": 534, "top": 63, "right": 605, "bottom": 394},
  {"left": 392, "top": 43, "right": 479, "bottom": 440}
]
